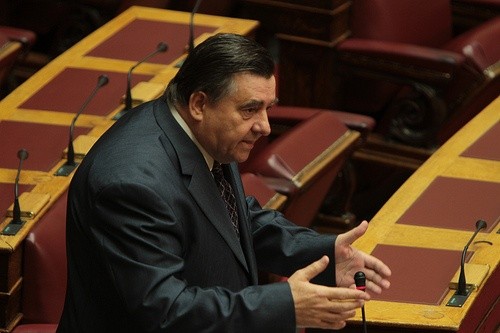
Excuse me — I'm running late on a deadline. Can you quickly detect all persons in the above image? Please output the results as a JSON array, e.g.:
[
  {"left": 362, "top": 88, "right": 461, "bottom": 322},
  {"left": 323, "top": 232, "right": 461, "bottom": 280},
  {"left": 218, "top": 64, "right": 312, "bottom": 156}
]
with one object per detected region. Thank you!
[{"left": 55, "top": 32, "right": 392, "bottom": 333}]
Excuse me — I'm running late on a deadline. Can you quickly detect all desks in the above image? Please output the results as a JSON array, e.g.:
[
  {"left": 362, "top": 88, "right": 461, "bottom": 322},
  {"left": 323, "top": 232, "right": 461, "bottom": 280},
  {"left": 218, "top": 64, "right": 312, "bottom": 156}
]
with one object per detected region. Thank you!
[
  {"left": 306, "top": 94, "right": 500, "bottom": 333},
  {"left": 0, "top": 4, "right": 259, "bottom": 332}
]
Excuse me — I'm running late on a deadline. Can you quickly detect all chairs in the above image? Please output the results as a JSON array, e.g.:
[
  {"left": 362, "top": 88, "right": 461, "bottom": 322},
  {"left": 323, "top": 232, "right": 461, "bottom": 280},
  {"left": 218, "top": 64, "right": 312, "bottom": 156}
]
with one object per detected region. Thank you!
[
  {"left": 336, "top": 0, "right": 500, "bottom": 145},
  {"left": 239, "top": 105, "right": 374, "bottom": 230},
  {"left": 1, "top": 25, "right": 37, "bottom": 84}
]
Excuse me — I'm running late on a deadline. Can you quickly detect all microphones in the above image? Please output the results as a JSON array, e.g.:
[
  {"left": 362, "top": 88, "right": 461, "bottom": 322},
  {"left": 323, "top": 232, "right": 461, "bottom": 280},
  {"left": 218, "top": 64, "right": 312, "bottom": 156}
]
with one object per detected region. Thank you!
[
  {"left": 67, "top": 74, "right": 110, "bottom": 164},
  {"left": 353, "top": 271, "right": 367, "bottom": 333},
  {"left": 125, "top": 41, "right": 168, "bottom": 110},
  {"left": 455, "top": 220, "right": 487, "bottom": 295},
  {"left": 13, "top": 149, "right": 29, "bottom": 224}
]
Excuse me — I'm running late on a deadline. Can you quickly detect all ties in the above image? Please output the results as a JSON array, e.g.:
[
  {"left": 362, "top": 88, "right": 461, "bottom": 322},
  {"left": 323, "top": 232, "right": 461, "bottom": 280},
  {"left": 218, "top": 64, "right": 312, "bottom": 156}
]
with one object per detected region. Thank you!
[{"left": 211, "top": 160, "right": 242, "bottom": 246}]
